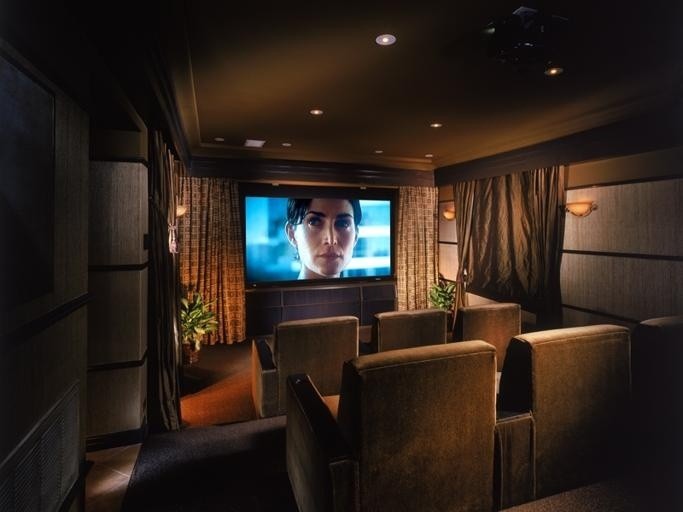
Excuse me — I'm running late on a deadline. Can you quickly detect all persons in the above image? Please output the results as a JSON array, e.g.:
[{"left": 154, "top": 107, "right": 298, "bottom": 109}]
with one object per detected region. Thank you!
[{"left": 284, "top": 197, "right": 363, "bottom": 280}]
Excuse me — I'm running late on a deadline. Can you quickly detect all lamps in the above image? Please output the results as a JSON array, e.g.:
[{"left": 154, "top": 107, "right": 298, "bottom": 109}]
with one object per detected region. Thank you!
[
  {"left": 441, "top": 206, "right": 456, "bottom": 221},
  {"left": 560, "top": 202, "right": 597, "bottom": 216},
  {"left": 175, "top": 204, "right": 186, "bottom": 217}
]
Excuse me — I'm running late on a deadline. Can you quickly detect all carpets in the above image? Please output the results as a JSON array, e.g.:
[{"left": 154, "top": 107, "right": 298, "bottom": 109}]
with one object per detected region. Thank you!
[{"left": 117, "top": 412, "right": 304, "bottom": 512}]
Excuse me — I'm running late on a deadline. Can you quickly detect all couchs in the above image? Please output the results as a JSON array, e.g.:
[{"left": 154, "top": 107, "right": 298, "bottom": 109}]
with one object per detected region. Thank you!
[
  {"left": 285, "top": 316, "right": 683, "bottom": 512},
  {"left": 252, "top": 302, "right": 522, "bottom": 419}
]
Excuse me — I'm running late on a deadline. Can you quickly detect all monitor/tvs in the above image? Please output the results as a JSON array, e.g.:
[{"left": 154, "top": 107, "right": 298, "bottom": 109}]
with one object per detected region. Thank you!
[{"left": 238, "top": 183, "right": 400, "bottom": 288}]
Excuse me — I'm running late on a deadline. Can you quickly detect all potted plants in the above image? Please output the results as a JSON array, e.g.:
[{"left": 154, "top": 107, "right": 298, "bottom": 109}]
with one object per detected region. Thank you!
[
  {"left": 173, "top": 292, "right": 219, "bottom": 372},
  {"left": 429, "top": 280, "right": 455, "bottom": 333}
]
